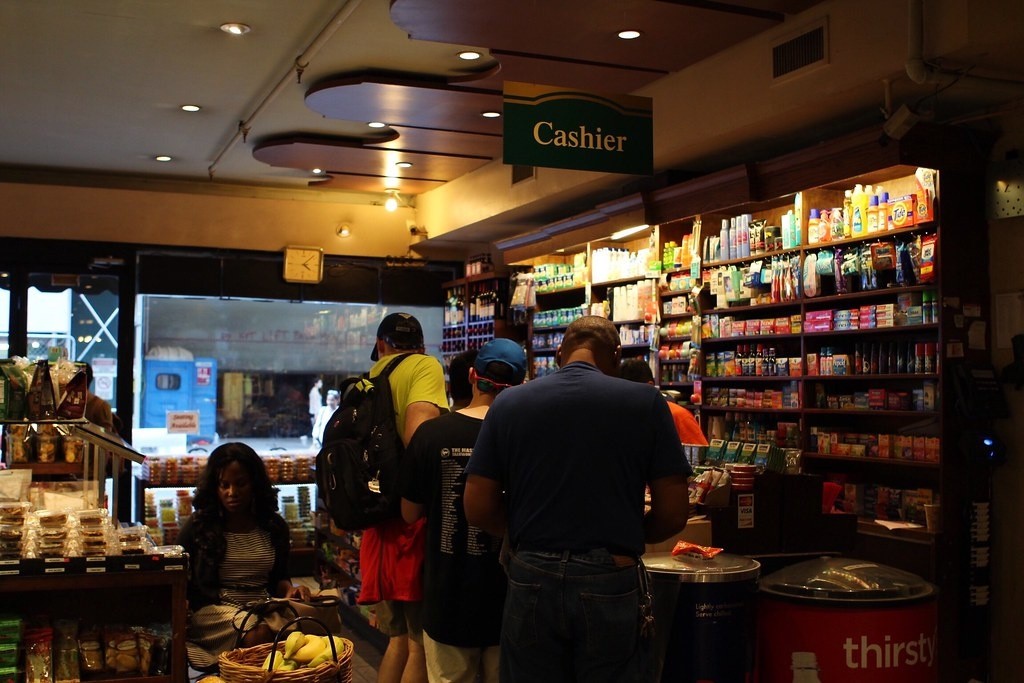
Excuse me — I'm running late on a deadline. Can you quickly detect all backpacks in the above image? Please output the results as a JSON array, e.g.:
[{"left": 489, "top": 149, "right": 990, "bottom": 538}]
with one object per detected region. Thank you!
[{"left": 314, "top": 349, "right": 424, "bottom": 530}]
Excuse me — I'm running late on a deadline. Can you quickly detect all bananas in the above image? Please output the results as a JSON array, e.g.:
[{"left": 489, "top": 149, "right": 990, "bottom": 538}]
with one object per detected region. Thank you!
[{"left": 261, "top": 630, "right": 345, "bottom": 667}]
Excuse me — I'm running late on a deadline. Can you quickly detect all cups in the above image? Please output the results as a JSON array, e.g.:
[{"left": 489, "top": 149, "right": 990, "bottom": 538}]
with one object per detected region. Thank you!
[{"left": 924, "top": 503, "right": 941, "bottom": 533}]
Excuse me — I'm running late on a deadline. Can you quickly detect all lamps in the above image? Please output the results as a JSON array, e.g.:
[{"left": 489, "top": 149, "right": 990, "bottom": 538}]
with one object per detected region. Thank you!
[{"left": 384, "top": 187, "right": 400, "bottom": 212}]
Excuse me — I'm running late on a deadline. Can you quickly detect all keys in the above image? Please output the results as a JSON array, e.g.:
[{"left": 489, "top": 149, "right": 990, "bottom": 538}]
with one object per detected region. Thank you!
[{"left": 640, "top": 616, "right": 655, "bottom": 640}]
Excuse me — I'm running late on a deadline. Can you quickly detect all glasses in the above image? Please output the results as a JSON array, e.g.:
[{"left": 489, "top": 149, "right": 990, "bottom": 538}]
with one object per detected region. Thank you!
[{"left": 474, "top": 369, "right": 513, "bottom": 395}]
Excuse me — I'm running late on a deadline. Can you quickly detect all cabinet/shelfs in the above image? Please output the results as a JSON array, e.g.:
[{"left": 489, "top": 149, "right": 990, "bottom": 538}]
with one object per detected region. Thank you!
[
  {"left": 654, "top": 164, "right": 968, "bottom": 683},
  {"left": 133, "top": 474, "right": 322, "bottom": 578},
  {"left": 441, "top": 272, "right": 525, "bottom": 356},
  {"left": 315, "top": 527, "right": 390, "bottom": 654},
  {"left": 0, "top": 553, "right": 191, "bottom": 683},
  {"left": 527, "top": 229, "right": 653, "bottom": 380}
]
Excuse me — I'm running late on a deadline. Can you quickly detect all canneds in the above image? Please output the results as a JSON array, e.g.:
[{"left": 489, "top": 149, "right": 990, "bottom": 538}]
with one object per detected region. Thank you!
[{"left": 532, "top": 308, "right": 582, "bottom": 379}]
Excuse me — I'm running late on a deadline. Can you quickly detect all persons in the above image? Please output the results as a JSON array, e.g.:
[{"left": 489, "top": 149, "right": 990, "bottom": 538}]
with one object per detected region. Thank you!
[
  {"left": 79, "top": 361, "right": 112, "bottom": 480},
  {"left": 617, "top": 358, "right": 655, "bottom": 386},
  {"left": 402, "top": 338, "right": 527, "bottom": 683},
  {"left": 175, "top": 443, "right": 341, "bottom": 672},
  {"left": 448, "top": 350, "right": 479, "bottom": 412},
  {"left": 464, "top": 317, "right": 693, "bottom": 683},
  {"left": 308, "top": 377, "right": 341, "bottom": 456},
  {"left": 357, "top": 313, "right": 451, "bottom": 683}
]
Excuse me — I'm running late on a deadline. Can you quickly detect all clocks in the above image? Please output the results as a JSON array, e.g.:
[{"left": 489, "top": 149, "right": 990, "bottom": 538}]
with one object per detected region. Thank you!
[{"left": 283, "top": 245, "right": 325, "bottom": 284}]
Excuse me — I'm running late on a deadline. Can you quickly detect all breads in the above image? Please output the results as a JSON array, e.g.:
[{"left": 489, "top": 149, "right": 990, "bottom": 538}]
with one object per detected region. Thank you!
[{"left": 80, "top": 646, "right": 151, "bottom": 676}]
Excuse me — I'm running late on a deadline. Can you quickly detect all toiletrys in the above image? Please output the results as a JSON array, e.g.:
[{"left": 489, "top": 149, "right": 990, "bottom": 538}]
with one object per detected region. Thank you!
[
  {"left": 662, "top": 233, "right": 694, "bottom": 269},
  {"left": 701, "top": 209, "right": 795, "bottom": 265},
  {"left": 592, "top": 246, "right": 656, "bottom": 345}
]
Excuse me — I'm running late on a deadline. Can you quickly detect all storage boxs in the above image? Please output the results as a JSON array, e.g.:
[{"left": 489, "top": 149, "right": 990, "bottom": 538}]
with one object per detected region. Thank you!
[
  {"left": 701, "top": 188, "right": 940, "bottom": 520},
  {"left": 0, "top": 617, "right": 26, "bottom": 683}
]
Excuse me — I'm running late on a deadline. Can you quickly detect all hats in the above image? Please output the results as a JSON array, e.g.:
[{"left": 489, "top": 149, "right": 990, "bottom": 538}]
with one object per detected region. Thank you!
[
  {"left": 472, "top": 338, "right": 527, "bottom": 382},
  {"left": 370, "top": 312, "right": 424, "bottom": 361}
]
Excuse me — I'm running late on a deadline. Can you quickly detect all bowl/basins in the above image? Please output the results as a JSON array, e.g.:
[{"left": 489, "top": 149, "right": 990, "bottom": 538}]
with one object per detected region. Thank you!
[{"left": 0, "top": 501, "right": 145, "bottom": 558}]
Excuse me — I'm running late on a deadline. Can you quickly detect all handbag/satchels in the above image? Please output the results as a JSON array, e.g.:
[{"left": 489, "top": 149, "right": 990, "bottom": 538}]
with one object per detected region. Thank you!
[{"left": 258, "top": 592, "right": 341, "bottom": 637}]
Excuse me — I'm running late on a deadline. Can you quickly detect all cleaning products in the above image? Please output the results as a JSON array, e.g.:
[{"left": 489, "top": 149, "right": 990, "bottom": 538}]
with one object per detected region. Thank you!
[{"left": 808, "top": 176, "right": 934, "bottom": 244}]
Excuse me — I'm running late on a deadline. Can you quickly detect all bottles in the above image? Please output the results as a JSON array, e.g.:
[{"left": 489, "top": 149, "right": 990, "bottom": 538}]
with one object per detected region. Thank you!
[
  {"left": 439, "top": 278, "right": 505, "bottom": 365},
  {"left": 807, "top": 183, "right": 889, "bottom": 245},
  {"left": 591, "top": 246, "right": 649, "bottom": 284},
  {"left": 720, "top": 214, "right": 751, "bottom": 259},
  {"left": 818, "top": 290, "right": 939, "bottom": 373},
  {"left": 734, "top": 343, "right": 776, "bottom": 376},
  {"left": 723, "top": 410, "right": 757, "bottom": 442}
]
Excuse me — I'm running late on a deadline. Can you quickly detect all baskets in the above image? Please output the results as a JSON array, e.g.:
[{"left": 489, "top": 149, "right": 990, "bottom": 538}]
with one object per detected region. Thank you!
[{"left": 218, "top": 614, "right": 353, "bottom": 683}]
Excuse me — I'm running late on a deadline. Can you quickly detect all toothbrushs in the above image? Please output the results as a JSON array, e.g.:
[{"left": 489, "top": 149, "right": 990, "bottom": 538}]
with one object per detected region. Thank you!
[{"left": 770, "top": 255, "right": 802, "bottom": 304}]
